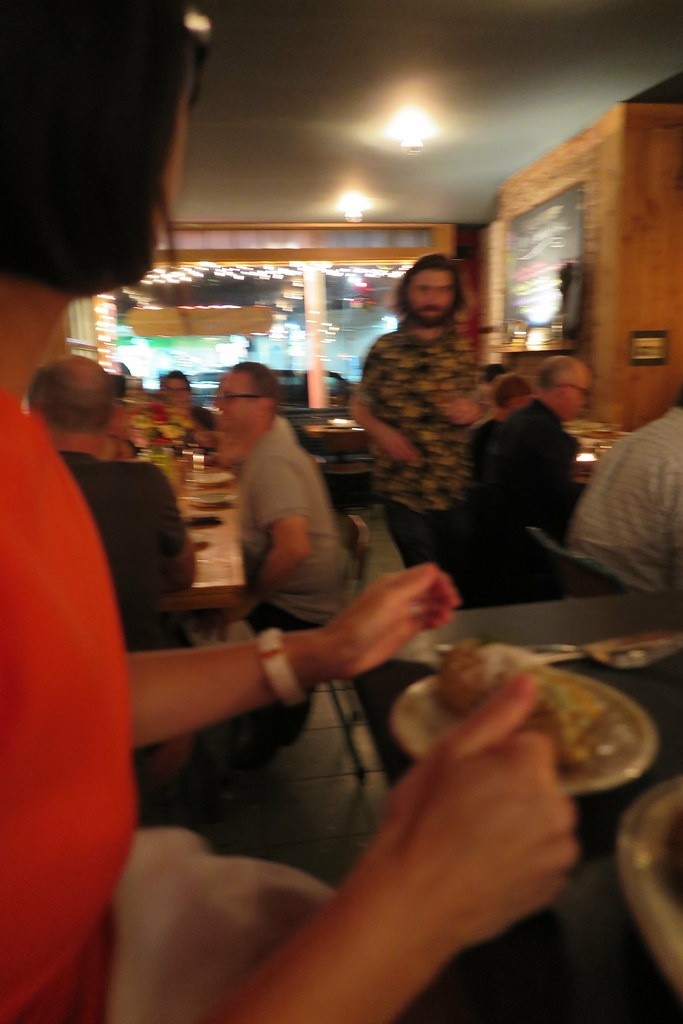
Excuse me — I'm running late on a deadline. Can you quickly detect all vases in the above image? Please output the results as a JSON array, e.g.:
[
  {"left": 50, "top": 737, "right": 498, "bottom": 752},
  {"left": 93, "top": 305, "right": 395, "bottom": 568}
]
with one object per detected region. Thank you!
[{"left": 137, "top": 449, "right": 175, "bottom": 476}]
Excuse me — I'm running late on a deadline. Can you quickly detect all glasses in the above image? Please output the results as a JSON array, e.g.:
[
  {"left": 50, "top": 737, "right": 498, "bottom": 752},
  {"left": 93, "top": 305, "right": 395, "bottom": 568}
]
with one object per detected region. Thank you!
[
  {"left": 213, "top": 390, "right": 269, "bottom": 402},
  {"left": 555, "top": 383, "right": 591, "bottom": 397}
]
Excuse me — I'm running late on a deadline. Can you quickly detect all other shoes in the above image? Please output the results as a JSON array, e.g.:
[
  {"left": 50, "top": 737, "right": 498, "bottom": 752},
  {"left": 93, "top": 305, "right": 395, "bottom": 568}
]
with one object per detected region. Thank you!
[{"left": 234, "top": 733, "right": 279, "bottom": 769}]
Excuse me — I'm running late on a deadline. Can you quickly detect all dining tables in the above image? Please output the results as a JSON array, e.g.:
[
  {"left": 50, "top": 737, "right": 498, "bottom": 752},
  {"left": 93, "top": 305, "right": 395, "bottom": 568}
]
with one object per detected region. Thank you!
[
  {"left": 361, "top": 590, "right": 683, "bottom": 1024},
  {"left": 301, "top": 420, "right": 373, "bottom": 473},
  {"left": 570, "top": 430, "right": 633, "bottom": 498},
  {"left": 152, "top": 463, "right": 248, "bottom": 611}
]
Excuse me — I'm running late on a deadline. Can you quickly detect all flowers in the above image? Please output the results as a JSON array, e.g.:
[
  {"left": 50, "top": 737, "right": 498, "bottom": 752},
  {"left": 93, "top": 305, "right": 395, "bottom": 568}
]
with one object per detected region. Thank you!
[{"left": 122, "top": 389, "right": 195, "bottom": 449}]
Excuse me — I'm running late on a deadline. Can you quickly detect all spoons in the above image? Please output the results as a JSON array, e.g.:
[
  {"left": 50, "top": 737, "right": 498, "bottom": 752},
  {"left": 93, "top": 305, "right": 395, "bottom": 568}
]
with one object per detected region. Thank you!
[{"left": 421, "top": 632, "right": 683, "bottom": 671}]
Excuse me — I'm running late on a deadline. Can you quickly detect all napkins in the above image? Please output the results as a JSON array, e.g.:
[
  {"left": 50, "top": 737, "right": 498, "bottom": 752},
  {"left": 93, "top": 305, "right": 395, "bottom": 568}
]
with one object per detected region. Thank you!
[{"left": 97, "top": 823, "right": 338, "bottom": 1024}]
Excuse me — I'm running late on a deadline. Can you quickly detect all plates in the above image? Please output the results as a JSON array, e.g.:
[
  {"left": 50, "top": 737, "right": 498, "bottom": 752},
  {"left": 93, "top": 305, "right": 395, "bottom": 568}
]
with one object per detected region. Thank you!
[
  {"left": 187, "top": 470, "right": 233, "bottom": 486},
  {"left": 190, "top": 492, "right": 238, "bottom": 508},
  {"left": 394, "top": 666, "right": 655, "bottom": 791}
]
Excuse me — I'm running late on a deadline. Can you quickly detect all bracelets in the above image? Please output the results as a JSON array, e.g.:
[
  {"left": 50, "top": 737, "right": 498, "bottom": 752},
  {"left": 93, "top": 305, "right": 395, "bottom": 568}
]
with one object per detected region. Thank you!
[{"left": 258, "top": 629, "right": 307, "bottom": 706}]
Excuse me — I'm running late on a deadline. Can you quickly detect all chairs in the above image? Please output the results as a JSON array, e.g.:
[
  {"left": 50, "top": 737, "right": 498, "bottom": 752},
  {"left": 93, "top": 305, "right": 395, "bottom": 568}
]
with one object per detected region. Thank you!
[
  {"left": 522, "top": 526, "right": 624, "bottom": 597},
  {"left": 321, "top": 514, "right": 372, "bottom": 786}
]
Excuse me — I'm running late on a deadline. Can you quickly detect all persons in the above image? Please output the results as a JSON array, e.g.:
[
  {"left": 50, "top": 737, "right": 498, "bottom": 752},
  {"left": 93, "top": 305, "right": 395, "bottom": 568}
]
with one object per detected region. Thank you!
[
  {"left": 34, "top": 353, "right": 357, "bottom": 653},
  {"left": 566, "top": 389, "right": 683, "bottom": 598},
  {"left": 468, "top": 376, "right": 531, "bottom": 488},
  {"left": 349, "top": 252, "right": 503, "bottom": 613},
  {"left": 1, "top": 0, "right": 581, "bottom": 1024},
  {"left": 495, "top": 356, "right": 589, "bottom": 600}
]
